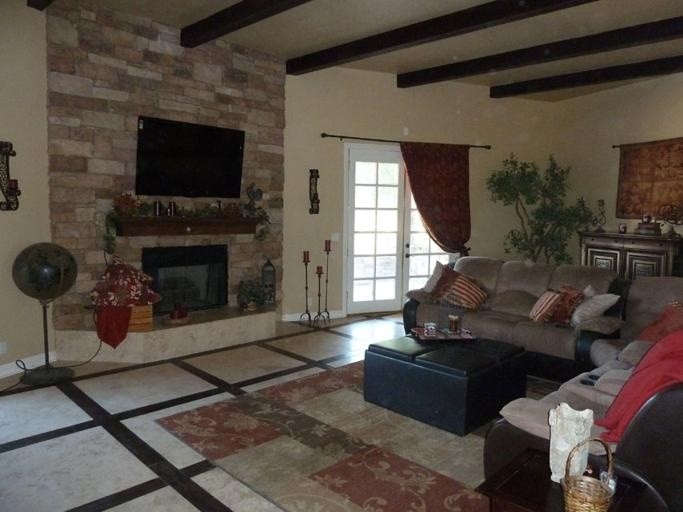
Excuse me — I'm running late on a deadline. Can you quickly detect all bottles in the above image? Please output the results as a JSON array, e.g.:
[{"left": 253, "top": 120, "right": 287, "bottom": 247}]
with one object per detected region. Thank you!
[
  {"left": 168, "top": 201, "right": 177, "bottom": 216},
  {"left": 153, "top": 200, "right": 161, "bottom": 215}
]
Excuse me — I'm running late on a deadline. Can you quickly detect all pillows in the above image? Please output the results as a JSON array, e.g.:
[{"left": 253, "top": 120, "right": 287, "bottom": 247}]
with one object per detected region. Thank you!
[
  {"left": 557, "top": 284, "right": 583, "bottom": 326},
  {"left": 445, "top": 274, "right": 488, "bottom": 313},
  {"left": 424, "top": 257, "right": 444, "bottom": 294},
  {"left": 526, "top": 289, "right": 567, "bottom": 324},
  {"left": 430, "top": 264, "right": 481, "bottom": 301},
  {"left": 569, "top": 283, "right": 622, "bottom": 329}
]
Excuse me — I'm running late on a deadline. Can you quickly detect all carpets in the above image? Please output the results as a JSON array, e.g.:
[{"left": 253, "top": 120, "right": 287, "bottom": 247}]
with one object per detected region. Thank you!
[{"left": 151, "top": 361, "right": 560, "bottom": 511}]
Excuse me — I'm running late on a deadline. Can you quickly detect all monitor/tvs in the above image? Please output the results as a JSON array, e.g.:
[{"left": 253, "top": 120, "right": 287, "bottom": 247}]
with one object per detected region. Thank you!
[{"left": 135, "top": 115, "right": 245, "bottom": 199}]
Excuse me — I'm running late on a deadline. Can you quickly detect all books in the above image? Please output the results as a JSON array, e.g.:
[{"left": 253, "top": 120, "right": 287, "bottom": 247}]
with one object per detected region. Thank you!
[
  {"left": 637, "top": 222, "right": 661, "bottom": 229},
  {"left": 633, "top": 228, "right": 661, "bottom": 235}
]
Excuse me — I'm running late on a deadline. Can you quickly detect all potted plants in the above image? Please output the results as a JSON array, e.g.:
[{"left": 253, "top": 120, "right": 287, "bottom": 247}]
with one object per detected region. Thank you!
[{"left": 237, "top": 279, "right": 265, "bottom": 311}]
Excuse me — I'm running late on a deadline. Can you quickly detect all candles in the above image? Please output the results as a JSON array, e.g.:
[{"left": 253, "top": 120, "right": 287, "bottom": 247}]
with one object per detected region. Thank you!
[{"left": 302, "top": 240, "right": 330, "bottom": 274}]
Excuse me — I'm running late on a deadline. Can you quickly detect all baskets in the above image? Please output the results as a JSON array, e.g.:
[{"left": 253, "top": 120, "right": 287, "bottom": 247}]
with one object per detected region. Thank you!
[{"left": 559, "top": 437, "right": 616, "bottom": 512}]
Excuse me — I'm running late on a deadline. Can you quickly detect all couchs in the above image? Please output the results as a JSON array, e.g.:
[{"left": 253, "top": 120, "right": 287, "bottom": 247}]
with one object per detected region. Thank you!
[{"left": 403, "top": 254, "right": 682, "bottom": 511}]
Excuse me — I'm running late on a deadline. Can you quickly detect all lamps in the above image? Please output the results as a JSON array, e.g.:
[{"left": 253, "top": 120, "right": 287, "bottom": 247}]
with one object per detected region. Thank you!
[{"left": 11, "top": 239, "right": 78, "bottom": 385}]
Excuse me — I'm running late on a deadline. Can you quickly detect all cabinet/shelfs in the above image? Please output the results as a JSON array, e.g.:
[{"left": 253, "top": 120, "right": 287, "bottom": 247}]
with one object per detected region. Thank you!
[{"left": 577, "top": 230, "right": 682, "bottom": 281}]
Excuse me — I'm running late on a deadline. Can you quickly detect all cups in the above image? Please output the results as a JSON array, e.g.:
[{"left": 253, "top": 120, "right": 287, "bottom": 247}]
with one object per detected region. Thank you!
[
  {"left": 598, "top": 466, "right": 617, "bottom": 494},
  {"left": 423, "top": 322, "right": 438, "bottom": 338},
  {"left": 448, "top": 315, "right": 459, "bottom": 332}
]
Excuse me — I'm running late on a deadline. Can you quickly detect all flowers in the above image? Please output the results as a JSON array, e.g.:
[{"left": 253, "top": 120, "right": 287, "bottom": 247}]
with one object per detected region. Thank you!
[{"left": 93, "top": 263, "right": 162, "bottom": 306}]
